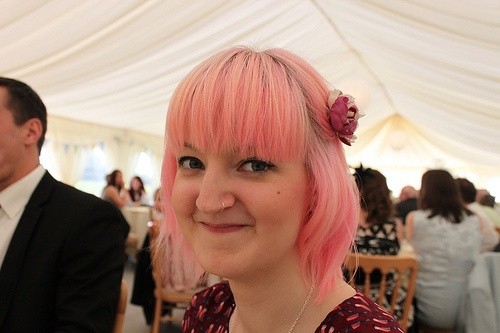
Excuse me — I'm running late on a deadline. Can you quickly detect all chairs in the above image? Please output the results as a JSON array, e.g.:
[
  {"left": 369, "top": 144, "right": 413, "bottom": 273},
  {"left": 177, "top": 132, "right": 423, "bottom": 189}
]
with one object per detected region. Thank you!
[
  {"left": 113, "top": 221, "right": 205, "bottom": 333},
  {"left": 342, "top": 252, "right": 418, "bottom": 333}
]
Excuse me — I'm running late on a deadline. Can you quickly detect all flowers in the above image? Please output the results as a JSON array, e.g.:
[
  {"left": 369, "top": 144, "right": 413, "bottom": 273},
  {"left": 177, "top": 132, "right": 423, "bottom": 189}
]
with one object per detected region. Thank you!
[{"left": 329, "top": 90, "right": 366, "bottom": 147}]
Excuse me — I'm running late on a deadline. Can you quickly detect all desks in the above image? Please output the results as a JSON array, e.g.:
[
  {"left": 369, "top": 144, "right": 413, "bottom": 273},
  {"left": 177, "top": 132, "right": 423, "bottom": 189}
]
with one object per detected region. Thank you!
[{"left": 119, "top": 206, "right": 152, "bottom": 249}]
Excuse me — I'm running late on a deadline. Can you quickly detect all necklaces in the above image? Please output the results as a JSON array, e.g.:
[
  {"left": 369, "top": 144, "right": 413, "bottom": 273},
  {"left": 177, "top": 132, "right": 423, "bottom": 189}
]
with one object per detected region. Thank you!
[{"left": 289, "top": 270, "right": 319, "bottom": 333}]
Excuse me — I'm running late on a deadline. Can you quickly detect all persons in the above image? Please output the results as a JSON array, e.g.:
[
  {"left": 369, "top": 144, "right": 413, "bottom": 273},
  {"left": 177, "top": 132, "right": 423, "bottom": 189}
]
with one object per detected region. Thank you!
[
  {"left": 342, "top": 164, "right": 500, "bottom": 333},
  {"left": 0, "top": 76, "right": 132, "bottom": 333},
  {"left": 160, "top": 45, "right": 404, "bottom": 333},
  {"left": 98, "top": 170, "right": 180, "bottom": 325}
]
